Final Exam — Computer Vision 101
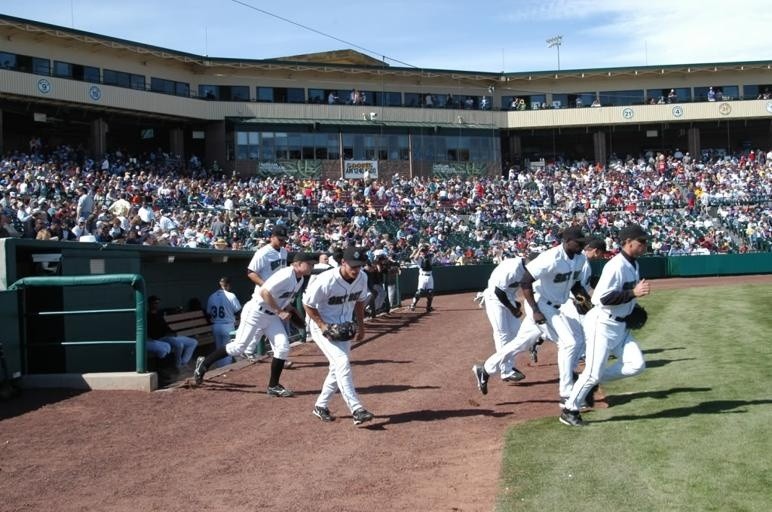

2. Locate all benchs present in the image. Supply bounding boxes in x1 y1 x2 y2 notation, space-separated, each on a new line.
148 309 240 352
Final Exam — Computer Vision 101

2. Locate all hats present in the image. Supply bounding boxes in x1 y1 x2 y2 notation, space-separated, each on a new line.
562 227 587 243
619 226 649 241
293 252 313 262
590 239 610 253
272 225 289 240
342 247 365 267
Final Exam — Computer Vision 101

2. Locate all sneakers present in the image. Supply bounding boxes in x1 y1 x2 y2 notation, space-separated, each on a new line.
558 397 588 412
502 370 522 382
353 408 373 425
473 364 489 395
411 303 415 309
312 406 332 421
558 408 584 427
529 337 544 363
266 384 293 397
426 308 432 313
194 356 207 383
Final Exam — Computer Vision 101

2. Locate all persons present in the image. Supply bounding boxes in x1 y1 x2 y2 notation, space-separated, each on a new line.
471 226 591 410
286 168 393 265
146 294 198 373
529 239 607 373
194 253 318 397
302 248 373 424
241 225 293 369
433 167 529 264
608 148 771 254
409 247 437 313
530 158 608 251
425 92 490 111
302 254 333 342
757 88 772 100
327 88 366 105
707 86 722 102
649 88 675 104
206 273 241 367
559 225 650 428
483 252 541 382
365 250 402 316
410 248 418 266
510 95 600 111
145 339 176 368
0 137 286 251
393 171 435 250
